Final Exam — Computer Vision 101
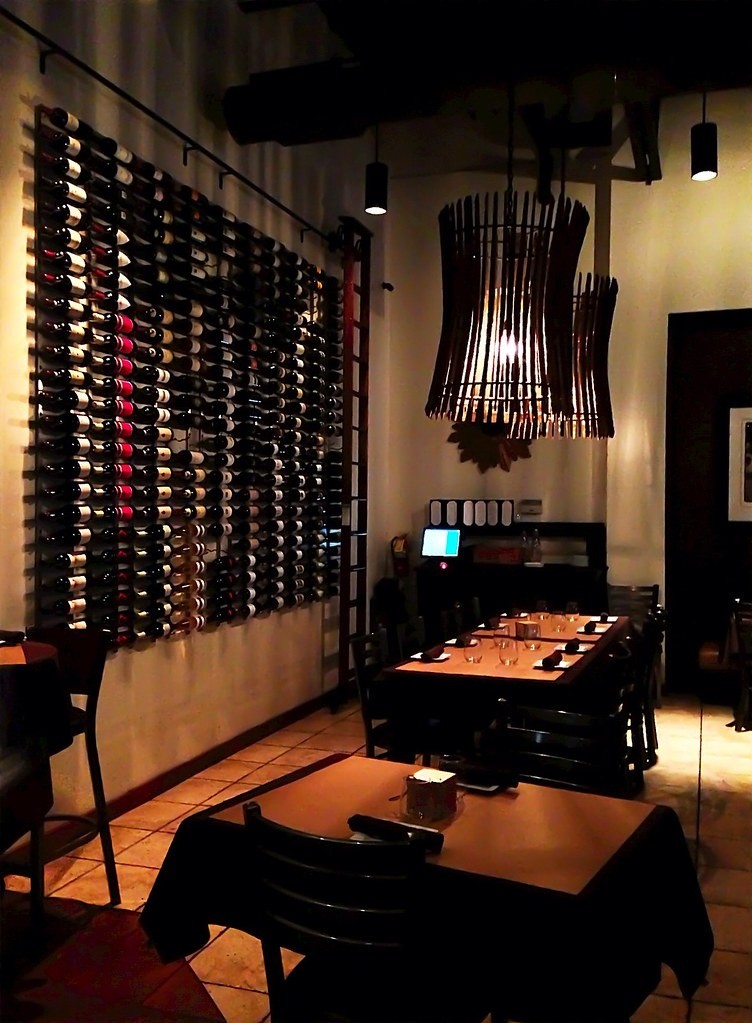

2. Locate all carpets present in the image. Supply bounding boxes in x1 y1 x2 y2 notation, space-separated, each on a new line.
0 885 227 1023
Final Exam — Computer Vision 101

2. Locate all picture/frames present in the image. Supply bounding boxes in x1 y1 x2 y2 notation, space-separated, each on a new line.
728 407 752 522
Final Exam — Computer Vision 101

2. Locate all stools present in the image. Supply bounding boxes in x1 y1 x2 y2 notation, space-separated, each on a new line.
1 627 118 918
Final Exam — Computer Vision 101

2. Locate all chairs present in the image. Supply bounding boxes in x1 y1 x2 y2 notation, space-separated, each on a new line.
350 584 669 801
242 802 488 1022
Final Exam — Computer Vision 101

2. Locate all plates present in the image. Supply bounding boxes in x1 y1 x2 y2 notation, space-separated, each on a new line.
533 659 570 668
590 616 618 622
445 639 477 645
411 652 451 660
553 644 587 652
500 613 527 617
478 623 508 628
577 626 606 633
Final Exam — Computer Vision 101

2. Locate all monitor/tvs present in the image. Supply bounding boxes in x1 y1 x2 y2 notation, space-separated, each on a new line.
420 527 461 559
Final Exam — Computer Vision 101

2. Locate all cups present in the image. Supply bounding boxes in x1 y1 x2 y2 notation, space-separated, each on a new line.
463 637 482 662
494 625 511 647
499 640 518 665
536 600 550 620
515 621 538 640
564 602 580 620
524 626 541 650
551 611 567 633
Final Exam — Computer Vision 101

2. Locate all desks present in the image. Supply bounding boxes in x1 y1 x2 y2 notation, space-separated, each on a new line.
720 599 752 732
0 642 77 936
139 753 717 1023
380 612 629 783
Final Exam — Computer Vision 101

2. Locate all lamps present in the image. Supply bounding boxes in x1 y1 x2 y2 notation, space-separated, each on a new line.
502 141 626 443
362 128 390 213
689 85 718 180
424 120 589 420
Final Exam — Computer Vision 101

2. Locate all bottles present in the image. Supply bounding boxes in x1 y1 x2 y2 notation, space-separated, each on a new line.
43 107 343 645
